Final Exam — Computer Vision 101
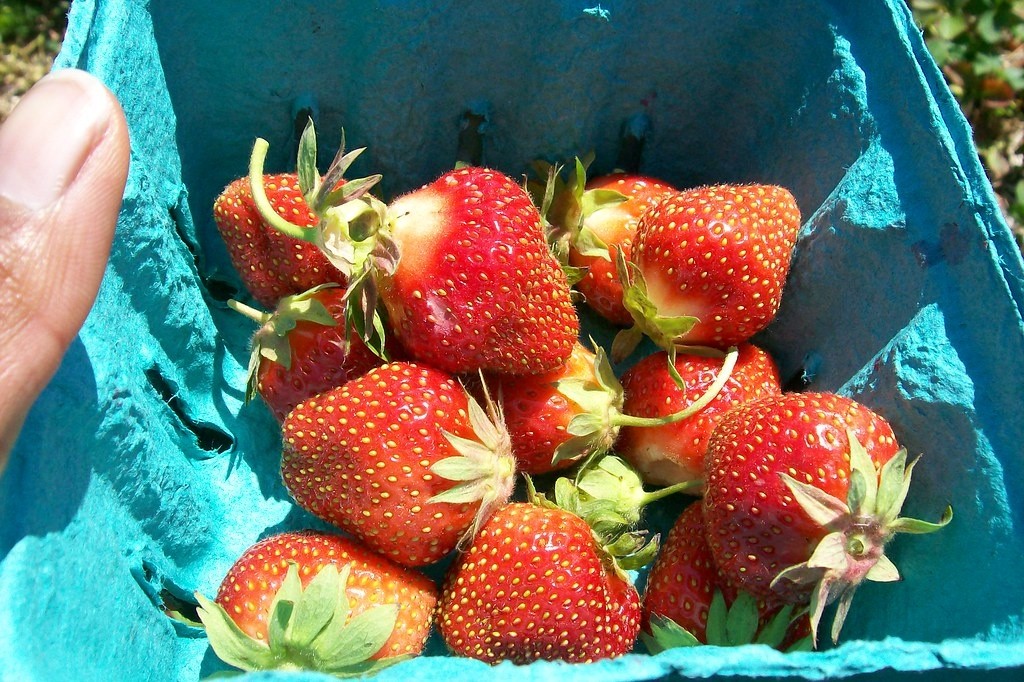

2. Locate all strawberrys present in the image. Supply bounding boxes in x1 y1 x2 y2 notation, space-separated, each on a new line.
187 116 956 681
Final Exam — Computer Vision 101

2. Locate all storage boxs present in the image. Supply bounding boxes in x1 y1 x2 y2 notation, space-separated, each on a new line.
0 0 1024 682
